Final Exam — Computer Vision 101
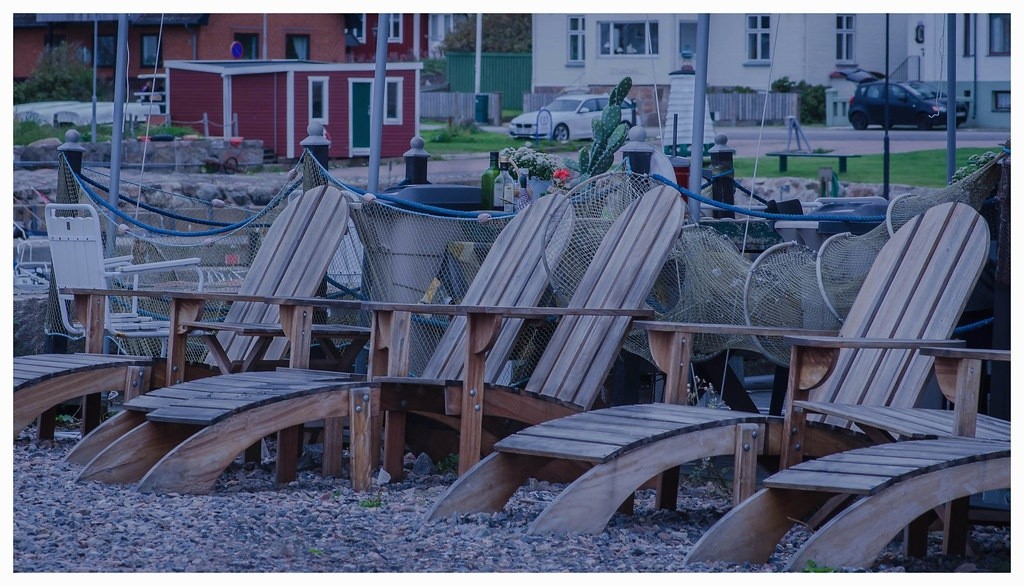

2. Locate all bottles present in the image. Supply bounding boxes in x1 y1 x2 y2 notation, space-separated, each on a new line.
515 172 531 213
480 151 500 210
492 162 514 214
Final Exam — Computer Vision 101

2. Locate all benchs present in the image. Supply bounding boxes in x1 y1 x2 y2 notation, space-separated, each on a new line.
765 147 863 174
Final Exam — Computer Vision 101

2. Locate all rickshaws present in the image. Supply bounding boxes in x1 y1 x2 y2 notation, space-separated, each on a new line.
200 152 247 176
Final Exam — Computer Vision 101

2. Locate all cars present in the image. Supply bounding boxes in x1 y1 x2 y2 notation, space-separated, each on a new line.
849 78 968 131
509 92 640 141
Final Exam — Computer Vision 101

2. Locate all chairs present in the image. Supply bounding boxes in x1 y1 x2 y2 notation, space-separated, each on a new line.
12 180 379 443
675 345 1012 574
421 199 996 539
42 201 211 357
59 191 576 488
137 180 690 506
